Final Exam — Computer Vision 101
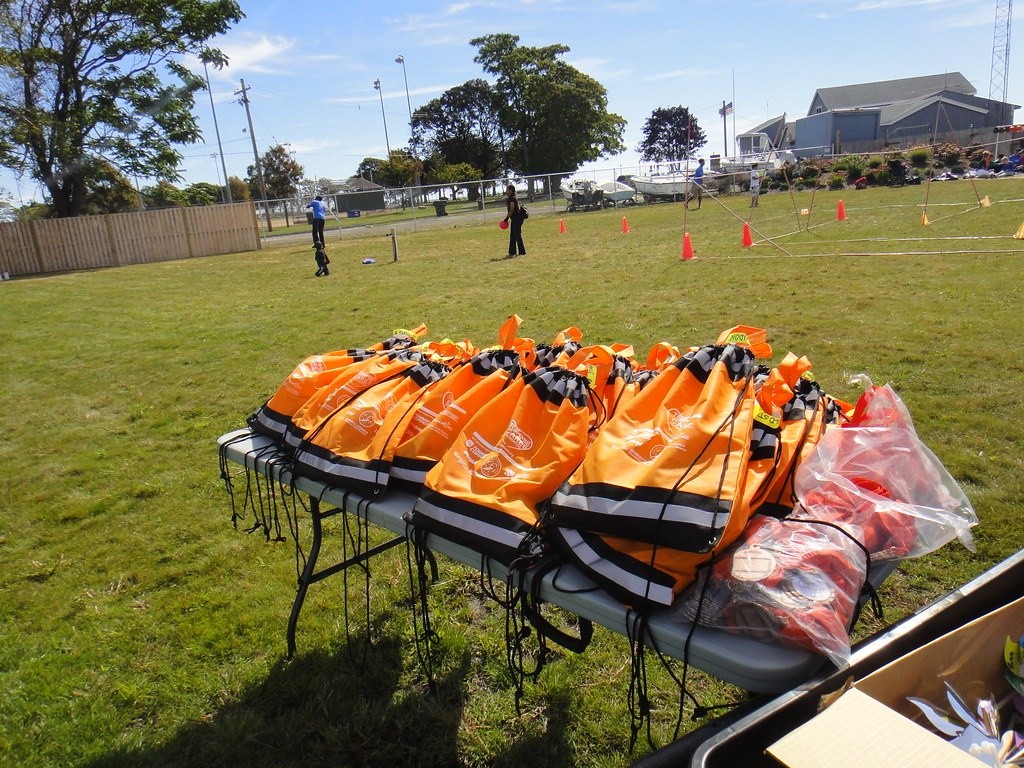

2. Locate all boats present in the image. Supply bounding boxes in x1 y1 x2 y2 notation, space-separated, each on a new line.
630 132 801 197
558 176 636 202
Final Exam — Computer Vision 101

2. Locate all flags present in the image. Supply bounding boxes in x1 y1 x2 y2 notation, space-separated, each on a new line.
721 102 732 118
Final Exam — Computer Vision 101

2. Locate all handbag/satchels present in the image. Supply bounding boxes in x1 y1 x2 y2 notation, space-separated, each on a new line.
248 325 846 615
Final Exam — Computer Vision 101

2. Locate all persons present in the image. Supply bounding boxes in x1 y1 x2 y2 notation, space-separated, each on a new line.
751 164 762 208
683 158 705 210
306 196 326 248
978 148 1024 179
501 185 527 258
315 241 330 277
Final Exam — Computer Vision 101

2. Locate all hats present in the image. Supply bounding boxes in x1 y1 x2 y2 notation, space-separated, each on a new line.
312 241 321 248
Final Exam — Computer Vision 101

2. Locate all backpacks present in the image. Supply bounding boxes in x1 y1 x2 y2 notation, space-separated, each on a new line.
509 197 529 220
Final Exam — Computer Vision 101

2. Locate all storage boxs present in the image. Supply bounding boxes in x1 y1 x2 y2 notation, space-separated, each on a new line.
690 545 1024 768
763 597 1024 768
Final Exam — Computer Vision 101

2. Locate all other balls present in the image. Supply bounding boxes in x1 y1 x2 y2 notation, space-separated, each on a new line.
500 221 509 230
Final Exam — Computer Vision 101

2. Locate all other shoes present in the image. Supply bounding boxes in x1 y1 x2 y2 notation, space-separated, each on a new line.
315 272 321 277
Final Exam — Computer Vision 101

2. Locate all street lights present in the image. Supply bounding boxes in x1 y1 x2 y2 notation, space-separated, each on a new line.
394 54 423 204
373 79 398 207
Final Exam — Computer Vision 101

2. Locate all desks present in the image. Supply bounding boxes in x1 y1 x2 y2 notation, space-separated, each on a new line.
217 427 896 702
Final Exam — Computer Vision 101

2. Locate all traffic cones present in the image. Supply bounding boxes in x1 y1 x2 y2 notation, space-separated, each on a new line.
834 198 849 222
619 216 630 234
559 218 567 234
741 222 757 248
679 232 697 261
